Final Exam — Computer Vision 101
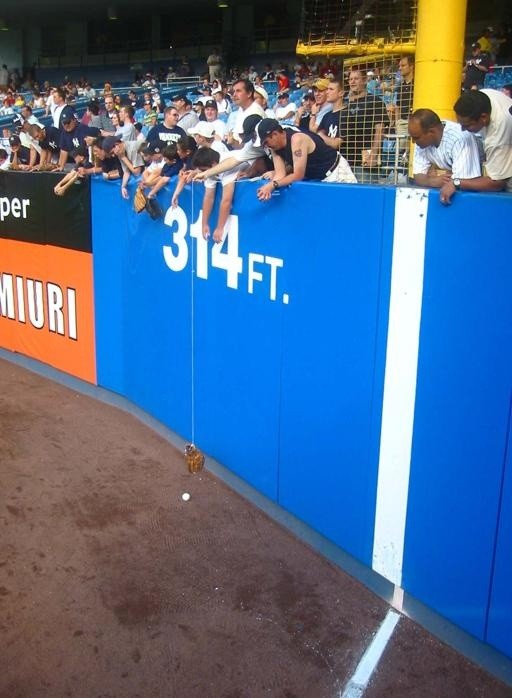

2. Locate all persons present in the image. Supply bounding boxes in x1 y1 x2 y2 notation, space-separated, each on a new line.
463 42 490 91
407 109 486 187
476 25 496 60
439 88 512 205
0 48 414 209
190 145 259 243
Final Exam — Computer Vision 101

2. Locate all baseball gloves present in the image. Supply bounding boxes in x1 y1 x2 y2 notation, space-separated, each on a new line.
39 162 59 171
146 197 160 220
185 444 205 475
9 162 26 170
133 188 146 214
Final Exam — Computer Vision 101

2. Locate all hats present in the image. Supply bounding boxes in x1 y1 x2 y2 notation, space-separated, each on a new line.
9 135 21 145
101 133 124 152
186 120 216 140
171 95 218 109
253 85 269 100
12 114 26 129
60 105 79 124
253 118 280 148
311 79 329 92
470 43 481 50
143 140 168 155
277 93 289 100
241 114 263 144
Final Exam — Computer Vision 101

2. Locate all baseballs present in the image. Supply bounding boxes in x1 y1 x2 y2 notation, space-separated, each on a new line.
181 493 191 500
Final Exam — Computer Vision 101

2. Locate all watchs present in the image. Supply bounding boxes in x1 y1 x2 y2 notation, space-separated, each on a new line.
453 178 461 190
